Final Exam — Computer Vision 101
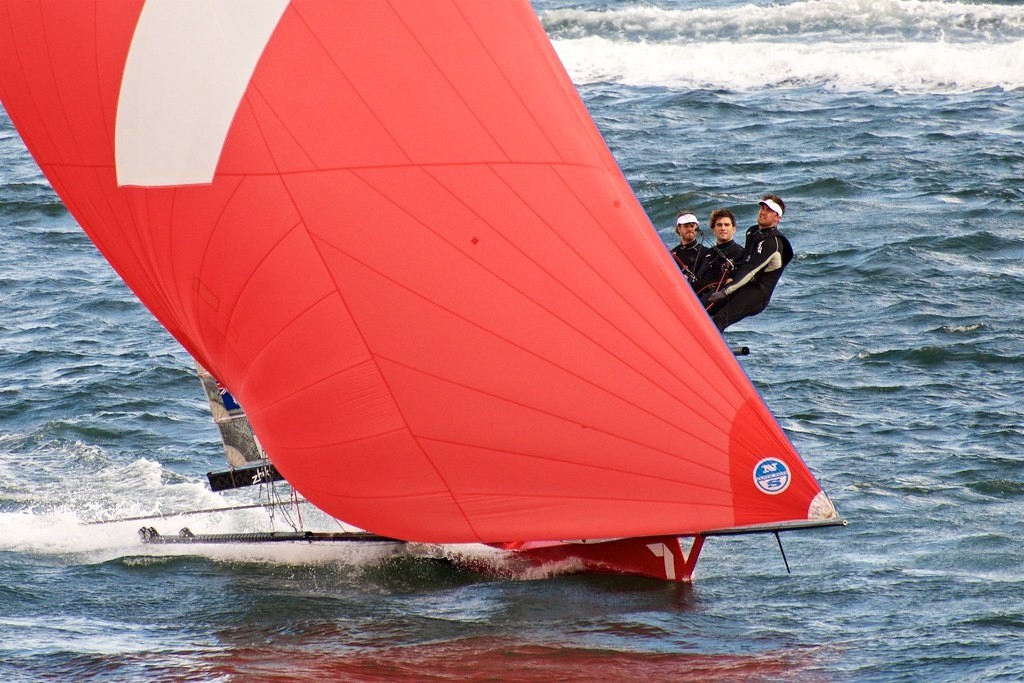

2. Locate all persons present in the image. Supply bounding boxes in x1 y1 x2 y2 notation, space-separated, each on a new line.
700 195 794 333
691 209 744 298
668 211 708 280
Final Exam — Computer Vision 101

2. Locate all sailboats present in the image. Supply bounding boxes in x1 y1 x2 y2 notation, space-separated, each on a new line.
0 0 848 592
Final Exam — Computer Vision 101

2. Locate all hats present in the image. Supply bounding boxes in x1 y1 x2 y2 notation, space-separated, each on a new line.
676 214 699 227
758 197 783 218
